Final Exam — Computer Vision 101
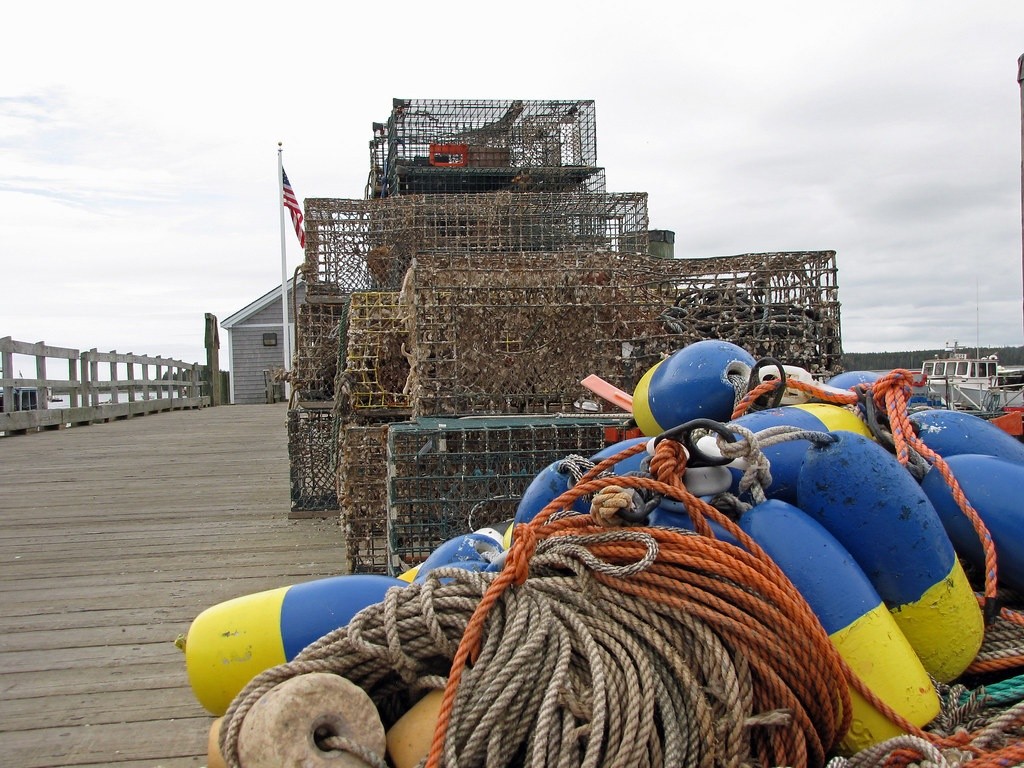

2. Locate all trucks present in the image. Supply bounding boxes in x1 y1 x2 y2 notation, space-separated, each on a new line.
0 386 49 412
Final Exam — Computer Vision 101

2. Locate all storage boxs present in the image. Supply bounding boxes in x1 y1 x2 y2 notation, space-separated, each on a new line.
283 97 842 574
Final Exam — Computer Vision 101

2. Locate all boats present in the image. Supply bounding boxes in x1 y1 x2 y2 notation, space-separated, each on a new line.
141 395 154 400
52 397 63 402
918 339 1024 409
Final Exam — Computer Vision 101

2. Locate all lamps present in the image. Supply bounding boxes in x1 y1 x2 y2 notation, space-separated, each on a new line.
263 333 278 346
